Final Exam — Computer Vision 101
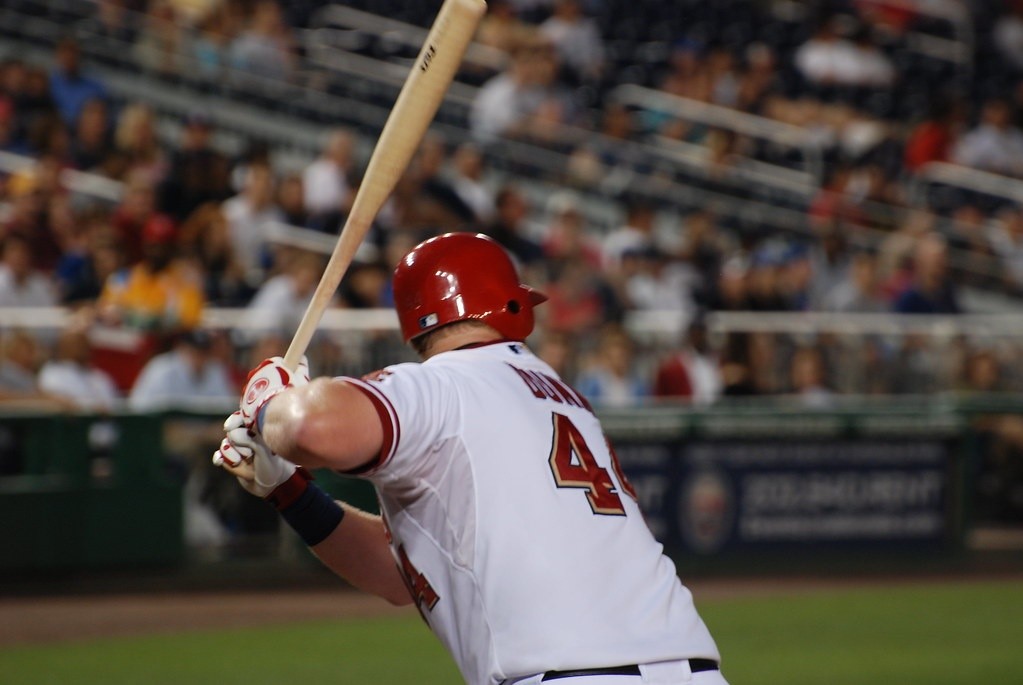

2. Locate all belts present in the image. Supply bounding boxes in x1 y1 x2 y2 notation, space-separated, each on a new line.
542 659 719 681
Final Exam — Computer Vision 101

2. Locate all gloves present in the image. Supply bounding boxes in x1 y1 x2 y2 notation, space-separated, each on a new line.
211 411 315 512
239 352 309 434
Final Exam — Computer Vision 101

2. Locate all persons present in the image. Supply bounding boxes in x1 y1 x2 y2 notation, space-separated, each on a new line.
0 0 1023 452
215 232 735 685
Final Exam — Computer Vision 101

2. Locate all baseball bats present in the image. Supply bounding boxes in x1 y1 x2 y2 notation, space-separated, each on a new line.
222 0 490 482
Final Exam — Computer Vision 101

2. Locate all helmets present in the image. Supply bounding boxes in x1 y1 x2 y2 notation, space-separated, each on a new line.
393 231 547 340
